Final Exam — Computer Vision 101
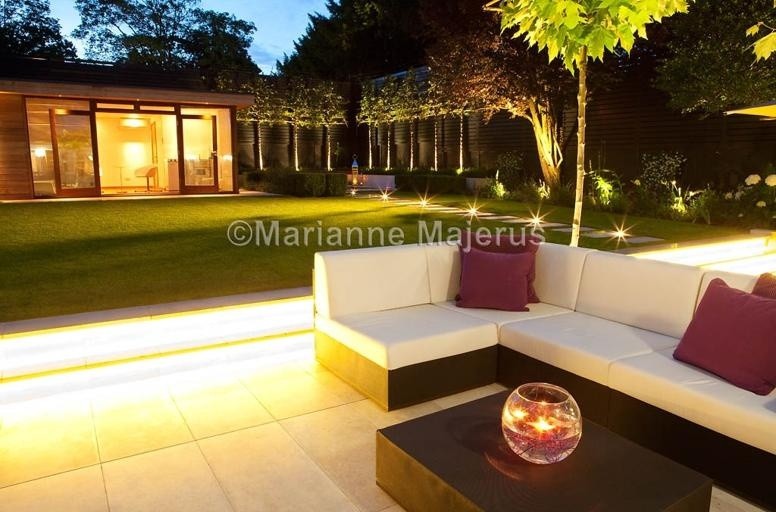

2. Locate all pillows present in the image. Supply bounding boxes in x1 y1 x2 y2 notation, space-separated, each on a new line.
455 230 541 303
673 279 776 395
751 273 776 298
456 244 534 312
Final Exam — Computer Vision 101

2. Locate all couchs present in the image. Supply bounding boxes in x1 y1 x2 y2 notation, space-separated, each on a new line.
427 239 776 512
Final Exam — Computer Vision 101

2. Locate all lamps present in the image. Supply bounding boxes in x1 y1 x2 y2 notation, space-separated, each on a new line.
351 154 359 185
501 382 583 465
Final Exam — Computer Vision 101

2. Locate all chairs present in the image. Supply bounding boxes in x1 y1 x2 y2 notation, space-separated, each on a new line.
134 165 162 192
313 244 498 412
